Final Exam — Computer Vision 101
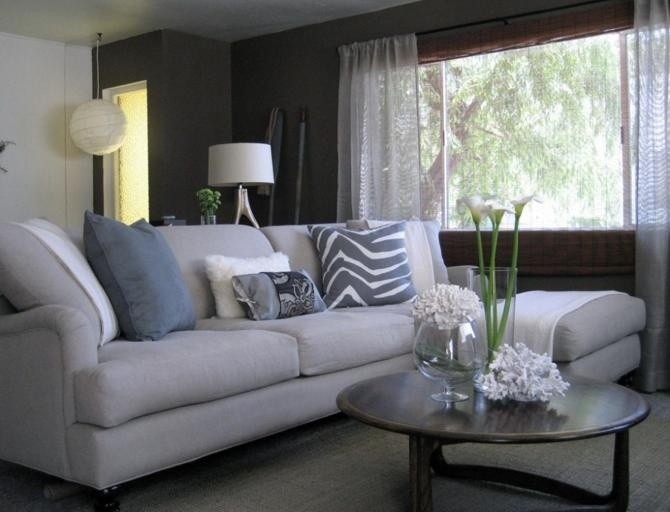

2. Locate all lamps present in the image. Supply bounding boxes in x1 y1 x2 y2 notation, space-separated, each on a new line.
67 30 130 158
209 143 275 228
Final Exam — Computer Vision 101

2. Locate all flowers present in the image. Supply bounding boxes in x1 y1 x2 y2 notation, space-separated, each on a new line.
194 187 221 224
457 192 545 373
474 342 570 406
410 281 480 333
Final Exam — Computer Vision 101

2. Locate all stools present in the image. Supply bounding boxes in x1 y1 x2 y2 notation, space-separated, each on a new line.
481 289 648 385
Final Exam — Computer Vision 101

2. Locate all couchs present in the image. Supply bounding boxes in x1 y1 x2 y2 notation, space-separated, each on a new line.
0 211 498 512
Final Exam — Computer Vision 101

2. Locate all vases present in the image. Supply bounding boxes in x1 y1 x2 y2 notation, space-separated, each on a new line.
506 389 537 403
467 268 517 390
200 215 217 225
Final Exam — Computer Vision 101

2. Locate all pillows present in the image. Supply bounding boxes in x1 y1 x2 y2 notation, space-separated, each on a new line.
84 212 198 340
200 251 291 319
0 215 120 350
231 269 328 320
306 222 419 310
